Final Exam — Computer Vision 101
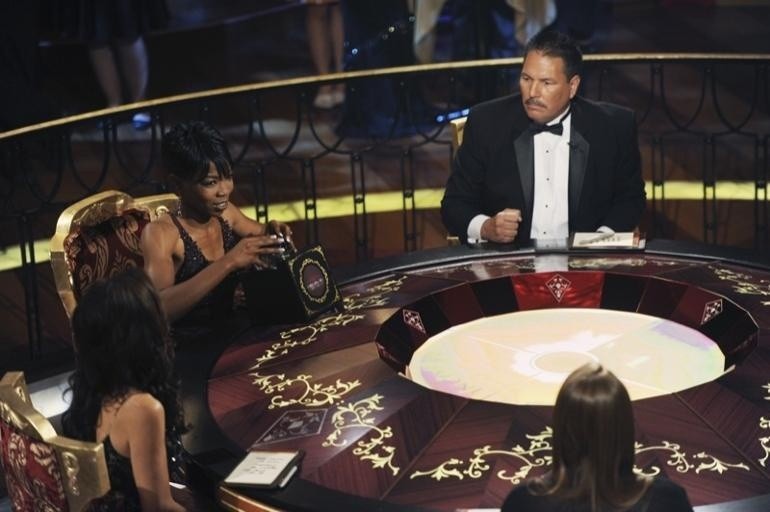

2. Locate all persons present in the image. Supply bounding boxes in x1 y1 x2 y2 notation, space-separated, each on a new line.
439 30 642 249
73 1 157 133
65 271 214 511
306 0 346 108
140 127 298 458
500 361 694 509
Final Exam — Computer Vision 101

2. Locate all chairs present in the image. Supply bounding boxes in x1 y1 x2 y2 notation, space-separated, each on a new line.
48 190 180 337
0 371 113 510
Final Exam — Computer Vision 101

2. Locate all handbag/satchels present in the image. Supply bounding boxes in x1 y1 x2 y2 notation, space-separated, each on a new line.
255 232 342 322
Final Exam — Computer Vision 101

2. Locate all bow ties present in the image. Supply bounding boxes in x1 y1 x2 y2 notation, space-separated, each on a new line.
528 121 564 137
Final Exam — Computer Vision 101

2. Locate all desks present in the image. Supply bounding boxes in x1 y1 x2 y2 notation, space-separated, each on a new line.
177 238 768 512
